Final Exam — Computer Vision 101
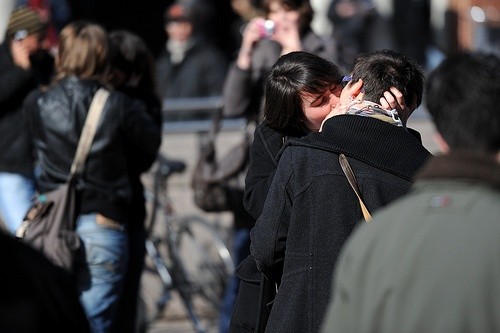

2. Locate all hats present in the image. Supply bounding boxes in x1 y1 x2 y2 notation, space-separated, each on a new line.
164 0 198 22
7 5 46 41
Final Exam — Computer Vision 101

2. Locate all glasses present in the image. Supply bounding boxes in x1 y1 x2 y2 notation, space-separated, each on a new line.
341 75 364 93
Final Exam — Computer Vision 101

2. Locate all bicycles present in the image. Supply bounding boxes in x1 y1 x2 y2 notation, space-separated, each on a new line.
141 153 237 333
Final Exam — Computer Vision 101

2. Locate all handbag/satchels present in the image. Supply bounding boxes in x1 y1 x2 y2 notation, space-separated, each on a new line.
192 105 254 212
14 171 83 275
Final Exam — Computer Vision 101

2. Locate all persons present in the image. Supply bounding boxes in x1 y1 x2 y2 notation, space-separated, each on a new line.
221 1 328 132
321 47 500 332
0 1 57 241
146 1 235 102
250 50 438 332
1 214 95 333
230 50 414 331
13 17 165 332
